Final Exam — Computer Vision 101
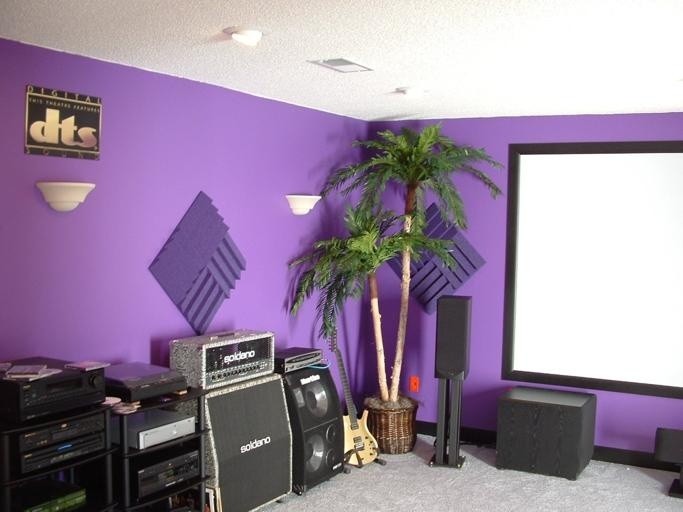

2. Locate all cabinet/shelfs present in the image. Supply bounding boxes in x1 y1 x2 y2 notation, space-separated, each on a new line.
0 386 212 512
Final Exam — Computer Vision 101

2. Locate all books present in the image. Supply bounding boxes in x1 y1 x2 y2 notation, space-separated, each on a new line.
1 368 63 382
195 483 222 512
4 363 47 378
63 358 111 371
165 481 213 512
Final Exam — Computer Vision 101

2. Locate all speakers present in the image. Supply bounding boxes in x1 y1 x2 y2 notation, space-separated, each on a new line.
436 294 472 376
202 375 293 512
283 361 344 494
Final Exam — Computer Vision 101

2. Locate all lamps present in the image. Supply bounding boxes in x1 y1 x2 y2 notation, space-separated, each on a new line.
36 182 96 212
285 195 322 215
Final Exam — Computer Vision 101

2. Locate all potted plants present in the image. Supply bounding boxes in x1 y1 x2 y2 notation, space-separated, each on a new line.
288 121 506 454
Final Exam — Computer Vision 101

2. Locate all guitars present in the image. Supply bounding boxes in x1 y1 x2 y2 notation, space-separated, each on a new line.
330 329 378 466
